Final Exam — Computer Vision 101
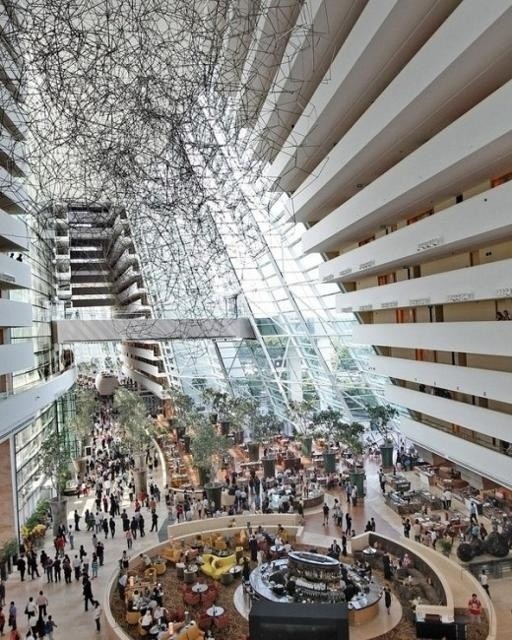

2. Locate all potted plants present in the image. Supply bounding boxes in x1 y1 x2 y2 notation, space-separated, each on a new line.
289 396 399 500
173 388 276 511
42 427 76 536
116 415 161 501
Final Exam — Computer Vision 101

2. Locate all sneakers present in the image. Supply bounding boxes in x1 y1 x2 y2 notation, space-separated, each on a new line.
322 522 329 526
91 575 98 580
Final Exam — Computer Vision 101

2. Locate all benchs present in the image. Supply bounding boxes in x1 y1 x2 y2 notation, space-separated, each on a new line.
360 546 441 607
198 550 239 583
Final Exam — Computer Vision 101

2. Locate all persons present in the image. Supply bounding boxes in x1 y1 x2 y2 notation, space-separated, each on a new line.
10 253 22 262
496 310 510 321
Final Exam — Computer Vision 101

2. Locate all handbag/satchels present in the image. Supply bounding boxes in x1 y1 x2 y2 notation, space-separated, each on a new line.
9 618 12 626
25 607 28 614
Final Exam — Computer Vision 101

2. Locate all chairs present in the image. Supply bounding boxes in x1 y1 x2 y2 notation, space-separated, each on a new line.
241 575 257 609
123 567 233 640
